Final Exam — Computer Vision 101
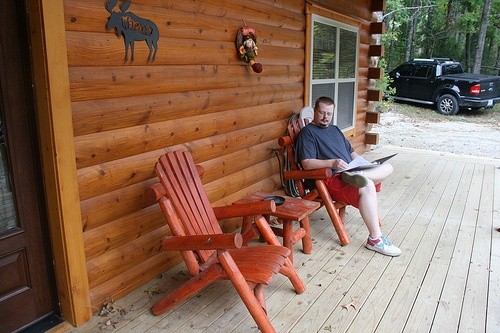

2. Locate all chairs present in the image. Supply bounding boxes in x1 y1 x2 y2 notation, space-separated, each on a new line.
277 117 383 246
146 149 306 332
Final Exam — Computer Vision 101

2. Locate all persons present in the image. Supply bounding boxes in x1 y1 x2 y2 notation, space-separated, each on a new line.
295 96 402 257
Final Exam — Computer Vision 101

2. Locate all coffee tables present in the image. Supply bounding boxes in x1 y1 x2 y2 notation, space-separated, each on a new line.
231 190 321 264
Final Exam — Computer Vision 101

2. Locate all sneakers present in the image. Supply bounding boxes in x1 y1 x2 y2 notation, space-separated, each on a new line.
365 234 402 256
342 170 369 189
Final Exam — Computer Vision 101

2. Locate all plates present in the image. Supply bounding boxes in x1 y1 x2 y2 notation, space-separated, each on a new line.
264 196 285 206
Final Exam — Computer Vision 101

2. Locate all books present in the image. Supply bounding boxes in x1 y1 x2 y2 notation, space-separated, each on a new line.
335 152 398 173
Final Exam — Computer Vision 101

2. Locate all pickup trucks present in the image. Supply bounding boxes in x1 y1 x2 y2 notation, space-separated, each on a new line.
384 58 500 115
